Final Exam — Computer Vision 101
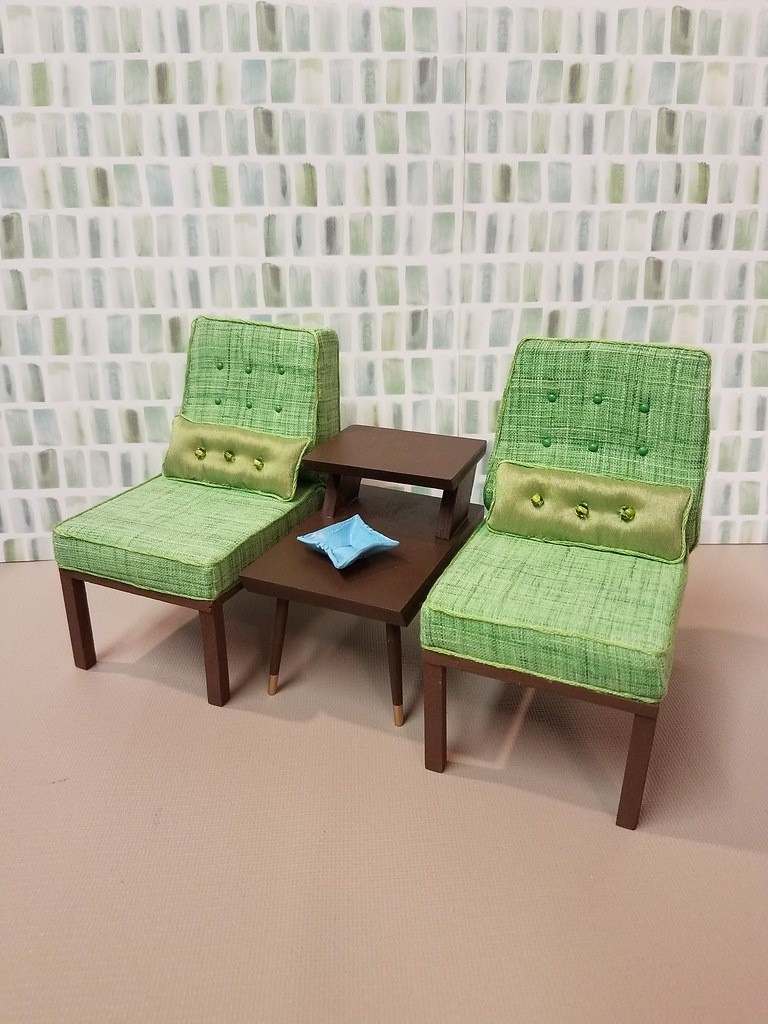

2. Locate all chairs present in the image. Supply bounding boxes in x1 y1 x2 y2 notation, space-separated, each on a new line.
417 333 715 832
48 311 345 710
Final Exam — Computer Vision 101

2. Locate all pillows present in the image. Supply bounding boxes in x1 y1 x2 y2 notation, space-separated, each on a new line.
486 457 694 566
162 410 314 504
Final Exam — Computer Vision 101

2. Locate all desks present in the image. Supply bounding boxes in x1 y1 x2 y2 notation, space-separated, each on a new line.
236 424 491 730
